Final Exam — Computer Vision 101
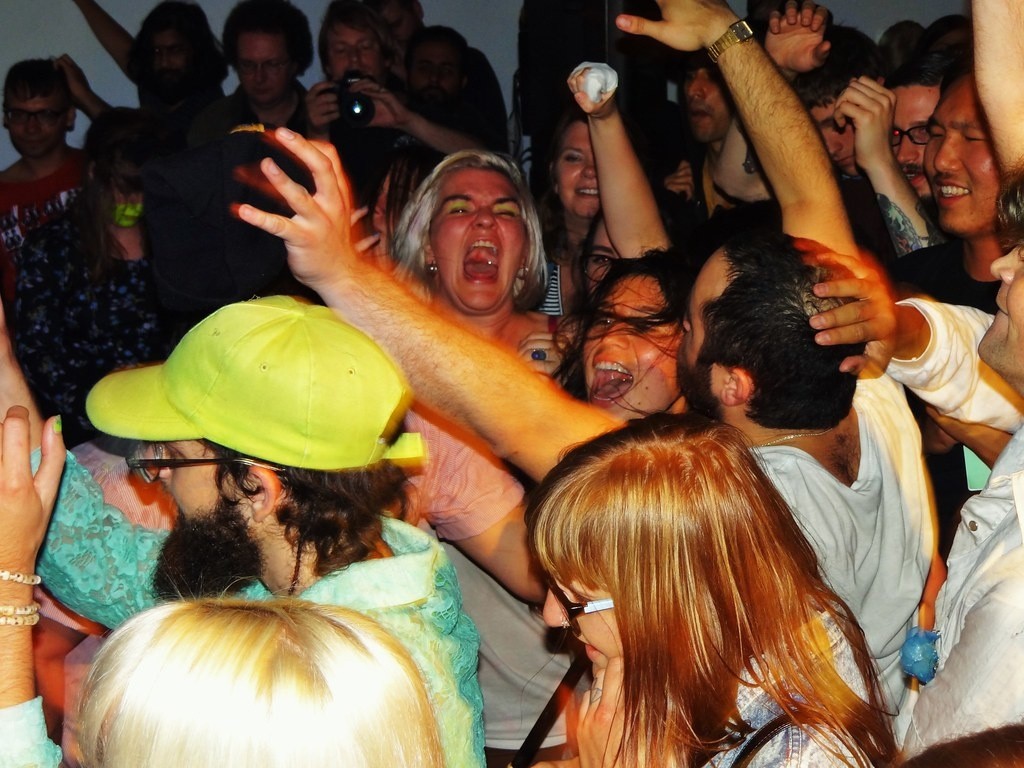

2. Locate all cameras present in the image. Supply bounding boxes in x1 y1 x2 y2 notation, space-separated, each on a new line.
317 69 382 128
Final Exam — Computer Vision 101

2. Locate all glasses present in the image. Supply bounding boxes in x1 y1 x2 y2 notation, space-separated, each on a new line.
120 440 281 484
576 252 615 282
2 105 66 128
889 121 934 151
543 574 616 622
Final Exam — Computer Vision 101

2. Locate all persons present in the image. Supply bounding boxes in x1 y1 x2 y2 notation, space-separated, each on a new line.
2 2 1024 767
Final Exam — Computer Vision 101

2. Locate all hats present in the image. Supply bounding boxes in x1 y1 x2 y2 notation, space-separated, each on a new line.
83 290 426 473
80 104 186 205
